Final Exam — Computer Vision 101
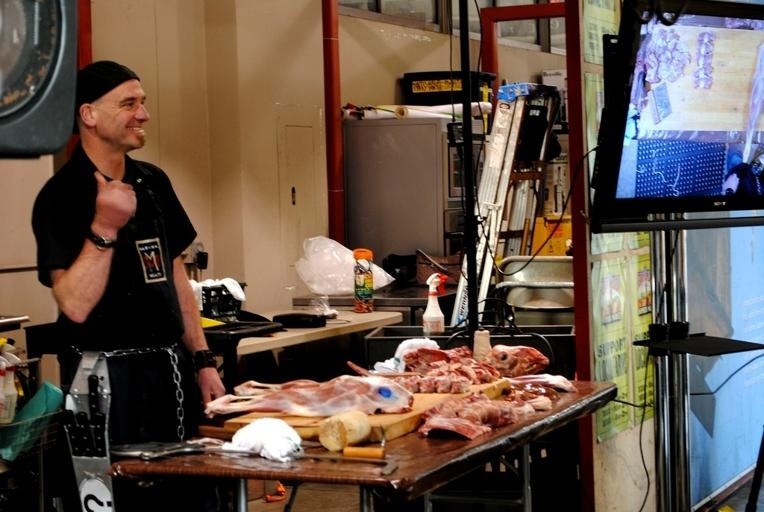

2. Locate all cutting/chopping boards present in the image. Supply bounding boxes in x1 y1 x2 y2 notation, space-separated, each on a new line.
224 379 511 441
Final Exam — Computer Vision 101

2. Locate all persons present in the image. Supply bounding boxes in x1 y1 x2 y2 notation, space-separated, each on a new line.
31 60 227 512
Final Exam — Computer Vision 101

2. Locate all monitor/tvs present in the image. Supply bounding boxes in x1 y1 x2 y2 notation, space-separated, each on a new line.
590 0 764 234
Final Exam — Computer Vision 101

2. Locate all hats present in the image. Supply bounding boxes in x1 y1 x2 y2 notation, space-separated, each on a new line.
74 62 140 133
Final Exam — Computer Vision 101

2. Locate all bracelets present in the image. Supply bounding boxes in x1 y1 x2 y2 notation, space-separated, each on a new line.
193 351 218 374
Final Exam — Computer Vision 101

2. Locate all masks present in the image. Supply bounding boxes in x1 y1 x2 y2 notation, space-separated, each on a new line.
222 416 302 463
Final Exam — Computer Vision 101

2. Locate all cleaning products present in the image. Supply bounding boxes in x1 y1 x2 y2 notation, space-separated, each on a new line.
0 338 20 424
423 272 448 332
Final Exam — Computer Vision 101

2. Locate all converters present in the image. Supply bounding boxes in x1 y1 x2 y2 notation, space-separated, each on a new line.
197 252 208 269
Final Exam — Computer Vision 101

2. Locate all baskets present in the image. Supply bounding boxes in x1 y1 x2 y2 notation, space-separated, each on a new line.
201 283 247 323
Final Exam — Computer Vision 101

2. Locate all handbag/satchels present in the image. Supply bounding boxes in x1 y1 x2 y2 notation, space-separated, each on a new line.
518 104 561 161
387 254 416 281
416 248 461 286
382 260 411 290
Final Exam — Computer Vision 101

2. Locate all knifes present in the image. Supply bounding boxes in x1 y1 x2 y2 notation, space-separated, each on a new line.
62 375 106 460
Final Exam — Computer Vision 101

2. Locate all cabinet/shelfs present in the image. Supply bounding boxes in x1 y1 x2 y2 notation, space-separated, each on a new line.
107 338 617 512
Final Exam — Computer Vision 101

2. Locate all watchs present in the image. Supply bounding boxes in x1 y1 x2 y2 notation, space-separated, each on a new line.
86 228 118 251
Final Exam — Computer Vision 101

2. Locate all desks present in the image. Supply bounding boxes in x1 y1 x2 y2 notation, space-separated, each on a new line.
204 308 403 392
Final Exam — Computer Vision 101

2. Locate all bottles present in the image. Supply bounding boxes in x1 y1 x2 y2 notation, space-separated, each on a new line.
354 249 374 313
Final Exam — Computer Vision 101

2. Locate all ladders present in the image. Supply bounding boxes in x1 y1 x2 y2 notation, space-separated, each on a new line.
450 83 556 328
